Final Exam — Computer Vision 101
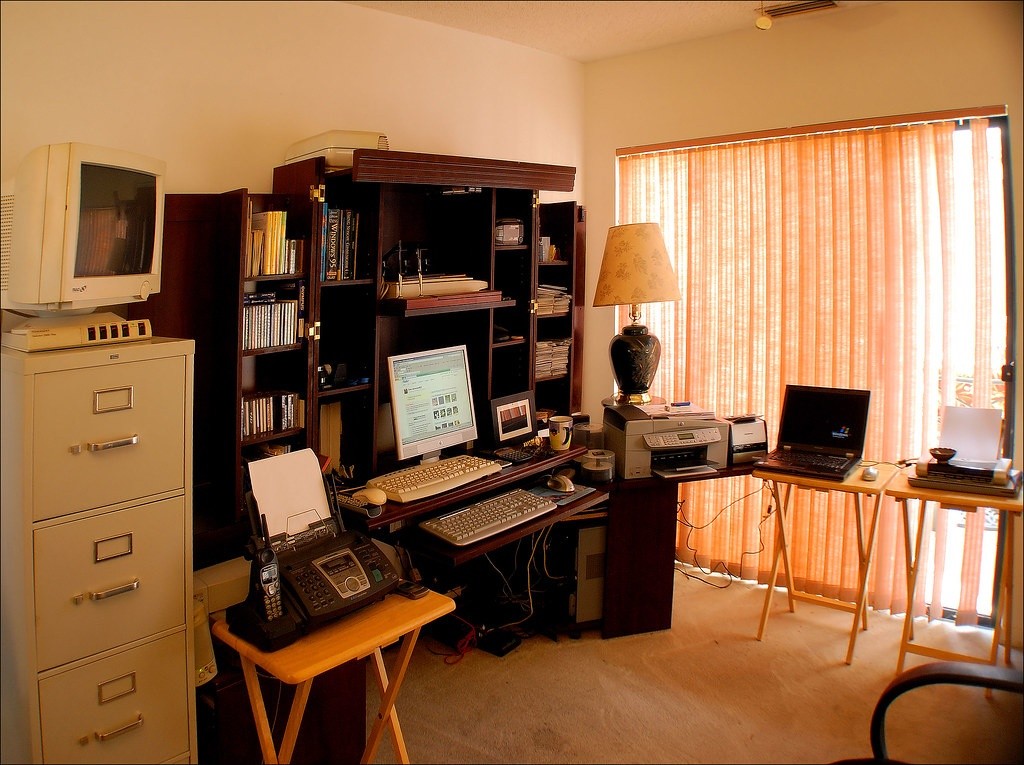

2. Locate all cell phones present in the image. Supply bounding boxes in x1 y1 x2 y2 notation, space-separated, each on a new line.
394 579 430 600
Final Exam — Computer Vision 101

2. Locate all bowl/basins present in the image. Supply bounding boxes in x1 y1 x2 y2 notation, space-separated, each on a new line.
929 448 957 463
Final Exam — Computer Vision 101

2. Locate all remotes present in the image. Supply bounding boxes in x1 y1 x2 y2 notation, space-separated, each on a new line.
330 494 383 518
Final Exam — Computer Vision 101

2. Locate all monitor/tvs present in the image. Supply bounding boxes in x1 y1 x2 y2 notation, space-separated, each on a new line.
0 141 166 353
387 345 478 465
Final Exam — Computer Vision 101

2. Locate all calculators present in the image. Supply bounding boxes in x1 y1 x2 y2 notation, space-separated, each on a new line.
493 447 533 463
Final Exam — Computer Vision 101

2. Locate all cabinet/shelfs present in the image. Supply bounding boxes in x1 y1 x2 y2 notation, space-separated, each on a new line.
601 464 757 639
202 145 585 565
0 335 199 765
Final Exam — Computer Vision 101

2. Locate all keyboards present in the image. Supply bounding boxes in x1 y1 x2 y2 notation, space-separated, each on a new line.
365 454 503 503
418 487 558 547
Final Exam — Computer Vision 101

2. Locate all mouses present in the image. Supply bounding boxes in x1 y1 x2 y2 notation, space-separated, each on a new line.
862 467 879 481
546 475 575 493
352 489 387 506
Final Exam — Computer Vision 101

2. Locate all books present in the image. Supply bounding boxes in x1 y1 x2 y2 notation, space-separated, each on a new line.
245 196 297 278
241 392 304 441
537 284 572 315
320 203 359 282
536 337 571 378
243 279 305 350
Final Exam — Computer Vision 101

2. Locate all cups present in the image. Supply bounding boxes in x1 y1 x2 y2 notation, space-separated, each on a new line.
571 412 590 425
549 416 573 450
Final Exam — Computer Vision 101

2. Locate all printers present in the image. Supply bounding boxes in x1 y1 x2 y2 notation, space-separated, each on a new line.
603 403 730 478
916 418 1013 486
716 413 768 466
244 475 399 634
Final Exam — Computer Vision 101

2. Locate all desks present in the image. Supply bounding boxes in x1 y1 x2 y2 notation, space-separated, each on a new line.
342 442 609 657
752 462 900 667
210 590 458 765
884 464 1024 698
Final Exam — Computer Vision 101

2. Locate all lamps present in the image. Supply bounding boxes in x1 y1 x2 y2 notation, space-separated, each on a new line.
593 221 685 407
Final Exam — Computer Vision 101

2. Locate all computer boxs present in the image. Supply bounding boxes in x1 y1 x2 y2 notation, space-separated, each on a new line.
546 525 603 624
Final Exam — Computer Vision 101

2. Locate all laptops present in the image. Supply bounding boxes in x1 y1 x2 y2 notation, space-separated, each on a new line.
752 385 870 480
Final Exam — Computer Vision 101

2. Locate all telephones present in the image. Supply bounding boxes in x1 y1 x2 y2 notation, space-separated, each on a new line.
224 514 296 653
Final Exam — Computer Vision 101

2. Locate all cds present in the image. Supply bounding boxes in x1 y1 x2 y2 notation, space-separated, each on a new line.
583 461 613 470
